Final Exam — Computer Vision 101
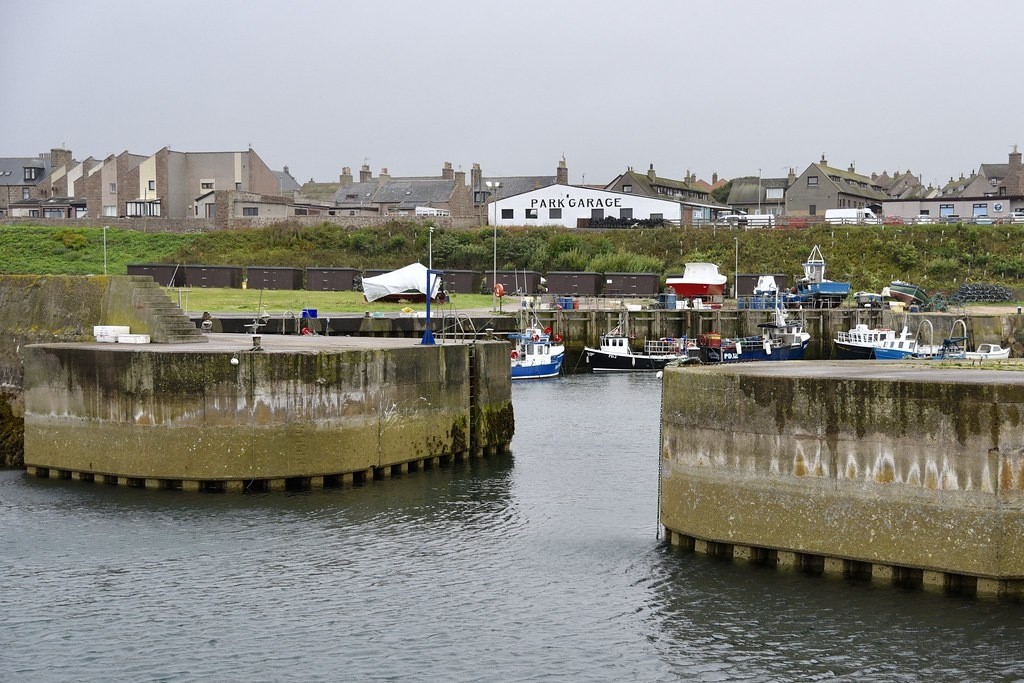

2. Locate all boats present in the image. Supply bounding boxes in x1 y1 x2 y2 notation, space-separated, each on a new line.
360 262 443 305
508 267 566 379
853 286 895 309
705 287 811 364
833 323 921 360
871 318 1014 360
889 280 930 306
736 275 802 310
794 243 851 307
584 304 704 374
665 262 728 310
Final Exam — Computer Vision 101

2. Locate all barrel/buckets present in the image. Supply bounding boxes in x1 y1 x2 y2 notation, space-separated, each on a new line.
557 298 579 310
739 297 782 309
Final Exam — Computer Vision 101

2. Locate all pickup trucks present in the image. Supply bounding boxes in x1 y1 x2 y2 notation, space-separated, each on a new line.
1002 208 1024 224
970 214 998 225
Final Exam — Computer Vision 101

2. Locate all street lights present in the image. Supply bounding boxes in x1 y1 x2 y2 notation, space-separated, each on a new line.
103 225 111 276
429 225 435 271
486 180 505 316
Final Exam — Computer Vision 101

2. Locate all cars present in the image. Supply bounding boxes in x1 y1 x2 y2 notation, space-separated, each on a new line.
938 214 963 226
884 215 904 225
909 214 936 225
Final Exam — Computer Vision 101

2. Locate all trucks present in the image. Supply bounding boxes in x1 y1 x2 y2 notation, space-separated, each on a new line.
825 207 883 225
714 214 776 230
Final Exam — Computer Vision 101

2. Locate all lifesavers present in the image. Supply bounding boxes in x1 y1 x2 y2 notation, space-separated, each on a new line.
688 342 696 347
495 284 503 297
699 335 707 346
881 328 891 331
